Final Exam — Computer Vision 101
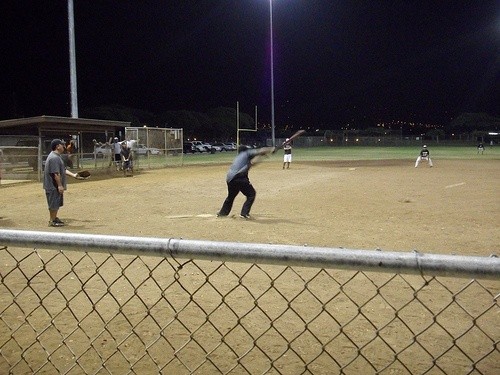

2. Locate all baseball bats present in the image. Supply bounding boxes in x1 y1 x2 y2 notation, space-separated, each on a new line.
273 129 304 153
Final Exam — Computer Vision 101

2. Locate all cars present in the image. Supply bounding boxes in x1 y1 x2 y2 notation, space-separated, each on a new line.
93 142 161 159
182 141 258 154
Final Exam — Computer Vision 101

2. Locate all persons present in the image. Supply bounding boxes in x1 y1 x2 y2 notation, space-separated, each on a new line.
283 139 292 169
415 145 432 168
477 143 485 154
217 146 275 218
43 136 140 226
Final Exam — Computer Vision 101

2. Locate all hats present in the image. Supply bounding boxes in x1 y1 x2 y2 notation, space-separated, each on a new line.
114 137 119 140
51 139 66 147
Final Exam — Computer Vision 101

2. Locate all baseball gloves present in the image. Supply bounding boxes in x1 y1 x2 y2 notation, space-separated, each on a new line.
74 170 92 180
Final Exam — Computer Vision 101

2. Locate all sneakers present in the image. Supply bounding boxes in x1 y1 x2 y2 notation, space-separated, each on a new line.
216 213 223 219
240 214 252 219
48 217 65 228
